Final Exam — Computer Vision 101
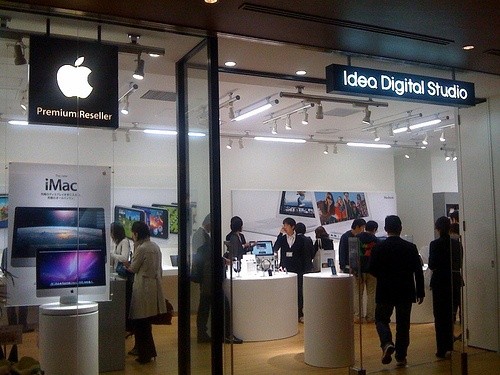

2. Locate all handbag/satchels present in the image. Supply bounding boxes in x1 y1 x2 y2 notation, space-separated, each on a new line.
313 238 322 272
150 299 173 325
115 261 132 275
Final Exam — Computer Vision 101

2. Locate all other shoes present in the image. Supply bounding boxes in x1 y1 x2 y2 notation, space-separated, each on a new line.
298 316 304 322
436 351 451 360
397 356 407 366
353 318 363 324
225 336 243 344
197 335 211 343
367 317 374 323
127 348 137 356
381 344 395 364
22 326 35 333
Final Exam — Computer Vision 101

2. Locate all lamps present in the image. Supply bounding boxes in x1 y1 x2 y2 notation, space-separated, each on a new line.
315 104 324 120
112 131 118 142
285 115 293 131
444 151 457 161
239 138 244 151
388 125 395 137
228 105 234 120
302 109 308 125
126 133 130 142
375 135 380 141
226 137 232 149
405 120 412 134
361 109 372 125
323 145 328 154
13 38 26 65
440 131 445 142
422 135 429 145
234 99 280 122
133 52 145 80
404 152 415 159
272 119 277 135
121 98 129 115
333 143 338 155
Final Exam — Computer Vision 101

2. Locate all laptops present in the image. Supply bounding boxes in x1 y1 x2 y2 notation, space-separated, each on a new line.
242 191 317 235
311 192 387 240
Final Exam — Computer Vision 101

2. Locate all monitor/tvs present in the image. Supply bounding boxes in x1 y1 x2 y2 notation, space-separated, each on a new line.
35 247 106 305
10 206 107 266
249 240 274 256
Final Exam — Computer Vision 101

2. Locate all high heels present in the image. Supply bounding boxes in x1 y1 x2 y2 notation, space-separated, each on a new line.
135 353 157 363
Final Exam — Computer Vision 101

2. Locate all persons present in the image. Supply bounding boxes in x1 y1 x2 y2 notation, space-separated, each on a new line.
317 192 368 226
429 216 465 359
225 216 258 260
364 221 381 322
296 191 307 207
110 221 134 338
339 219 369 324
370 215 426 367
123 220 173 365
313 226 335 268
190 213 244 344
0 247 35 333
273 218 315 323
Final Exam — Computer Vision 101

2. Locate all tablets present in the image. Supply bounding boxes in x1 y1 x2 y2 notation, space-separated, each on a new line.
170 255 178 266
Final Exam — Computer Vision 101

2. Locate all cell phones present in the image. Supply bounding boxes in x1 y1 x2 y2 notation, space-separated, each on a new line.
330 266 337 276
114 204 179 241
268 269 272 277
